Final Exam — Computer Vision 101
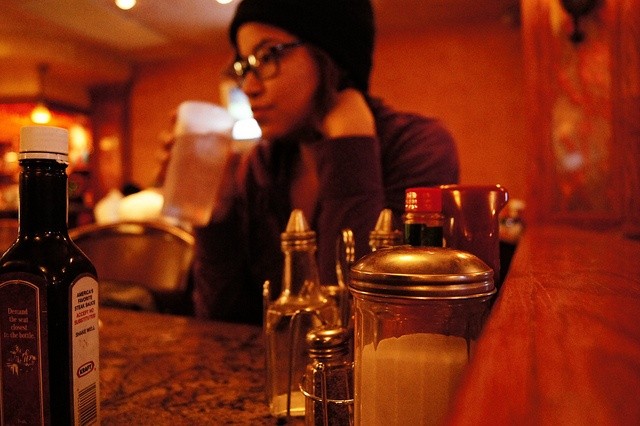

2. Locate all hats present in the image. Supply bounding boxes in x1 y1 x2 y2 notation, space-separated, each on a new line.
229 0 375 89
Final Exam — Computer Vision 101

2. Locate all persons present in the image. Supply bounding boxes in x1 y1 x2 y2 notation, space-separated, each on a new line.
190 0 460 327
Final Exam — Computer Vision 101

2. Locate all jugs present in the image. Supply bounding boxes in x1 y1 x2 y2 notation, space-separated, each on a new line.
344 245 498 426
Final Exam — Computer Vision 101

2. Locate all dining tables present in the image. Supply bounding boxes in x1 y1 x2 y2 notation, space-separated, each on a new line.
98 305 272 426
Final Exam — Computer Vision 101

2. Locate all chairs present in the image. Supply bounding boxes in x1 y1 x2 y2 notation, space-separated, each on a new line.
70 218 196 316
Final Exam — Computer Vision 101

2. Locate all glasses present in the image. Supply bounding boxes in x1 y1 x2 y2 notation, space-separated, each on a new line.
227 36 301 81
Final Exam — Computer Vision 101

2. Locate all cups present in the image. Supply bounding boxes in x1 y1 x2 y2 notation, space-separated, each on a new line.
160 101 237 225
438 186 508 282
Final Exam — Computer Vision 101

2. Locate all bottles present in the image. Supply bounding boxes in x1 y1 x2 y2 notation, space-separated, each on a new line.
267 209 336 418
370 209 402 251
405 189 443 247
0 127 99 426
304 325 354 426
